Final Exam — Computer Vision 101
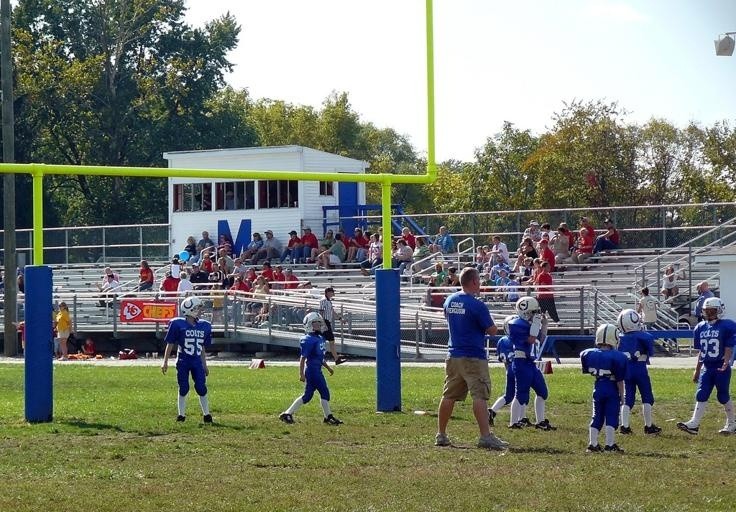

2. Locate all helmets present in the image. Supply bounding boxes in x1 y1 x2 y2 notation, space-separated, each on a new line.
701 297 726 321
595 323 621 349
303 312 328 332
515 297 541 321
503 314 518 338
180 296 205 320
617 309 645 332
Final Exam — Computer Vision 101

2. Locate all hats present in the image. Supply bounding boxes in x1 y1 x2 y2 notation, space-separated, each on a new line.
192 263 199 266
530 216 613 244
325 287 336 292
264 225 311 235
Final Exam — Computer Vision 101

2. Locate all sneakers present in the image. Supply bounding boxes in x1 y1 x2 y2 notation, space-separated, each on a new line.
278 412 295 424
336 358 347 366
323 414 343 425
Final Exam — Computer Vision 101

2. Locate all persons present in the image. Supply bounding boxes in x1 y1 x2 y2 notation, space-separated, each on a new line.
507 294 558 431
277 310 345 426
675 296 736 437
225 191 240 209
662 266 687 299
638 286 661 330
580 322 629 453
158 225 320 328
0 259 156 361
487 313 536 427
310 226 454 284
424 217 621 325
434 266 511 449
695 282 702 295
159 295 213 424
615 308 664 435
317 288 347 365
194 191 211 209
694 279 716 323
